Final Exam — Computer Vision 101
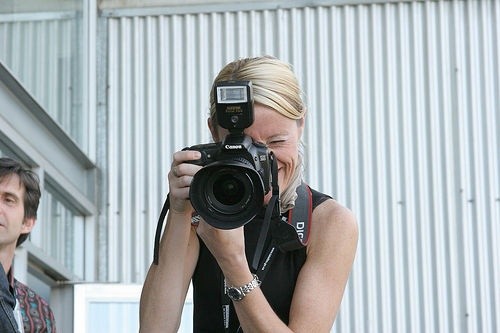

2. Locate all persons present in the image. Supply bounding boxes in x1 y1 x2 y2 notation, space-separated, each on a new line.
136 53 360 332
0 156 59 333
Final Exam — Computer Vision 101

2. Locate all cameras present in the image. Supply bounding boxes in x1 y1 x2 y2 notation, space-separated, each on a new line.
180 80 273 231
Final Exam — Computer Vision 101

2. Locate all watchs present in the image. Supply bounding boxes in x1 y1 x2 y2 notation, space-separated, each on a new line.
225 272 261 300
